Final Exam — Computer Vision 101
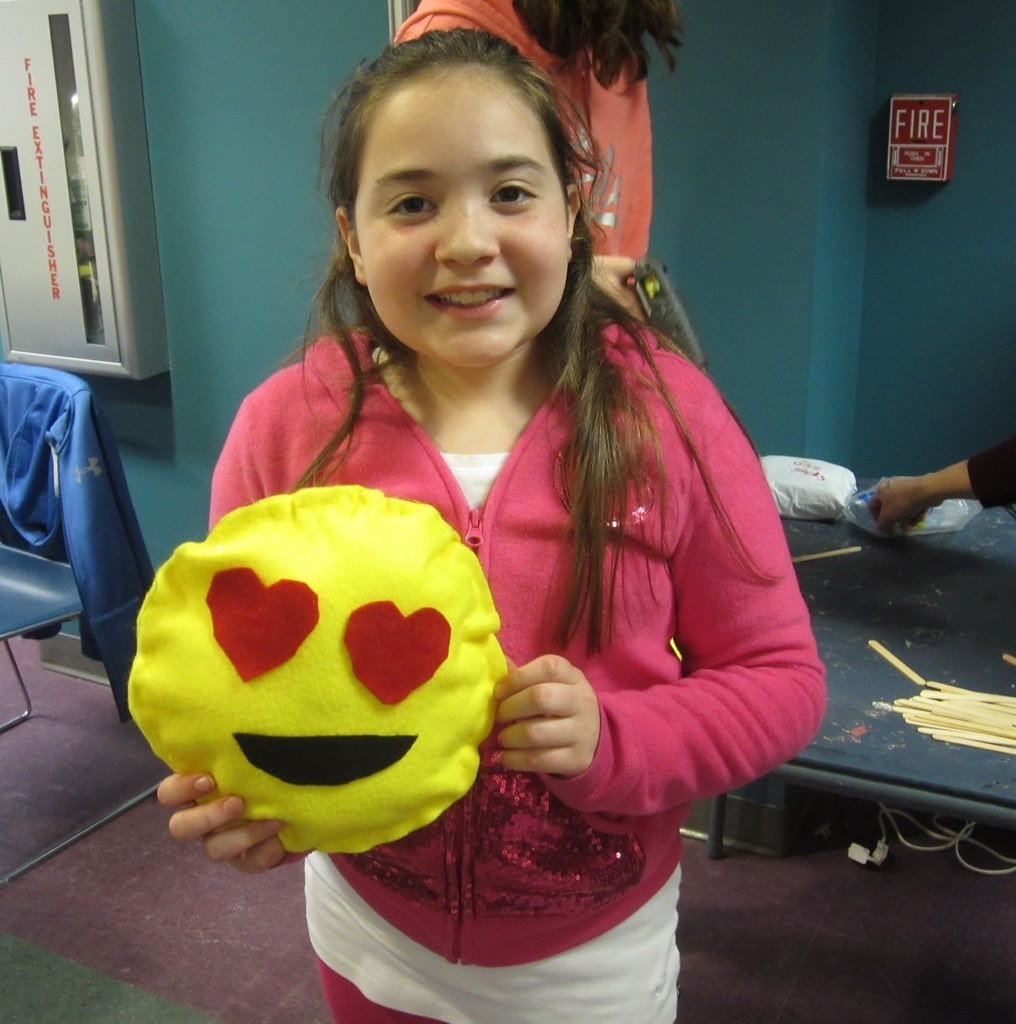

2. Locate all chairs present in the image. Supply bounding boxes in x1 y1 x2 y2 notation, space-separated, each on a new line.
0 362 157 737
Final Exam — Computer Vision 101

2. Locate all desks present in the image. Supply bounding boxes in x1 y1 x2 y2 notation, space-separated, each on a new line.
704 456 1016 857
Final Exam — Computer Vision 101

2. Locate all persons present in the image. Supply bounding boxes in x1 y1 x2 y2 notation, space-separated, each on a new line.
158 28 828 1023
392 0 683 324
867 436 1016 534
